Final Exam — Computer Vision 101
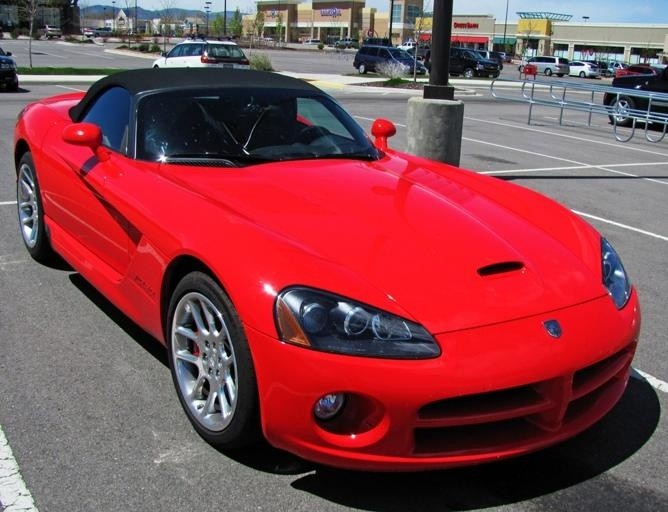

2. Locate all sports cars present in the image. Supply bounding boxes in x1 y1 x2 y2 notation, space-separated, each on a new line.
12 67 640 481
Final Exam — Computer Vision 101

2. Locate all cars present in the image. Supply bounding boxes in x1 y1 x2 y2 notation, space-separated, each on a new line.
353 41 429 74
335 38 359 48
40 24 62 38
0 46 19 92
151 40 251 70
604 61 667 132
426 47 503 78
83 26 110 36
520 55 599 78
